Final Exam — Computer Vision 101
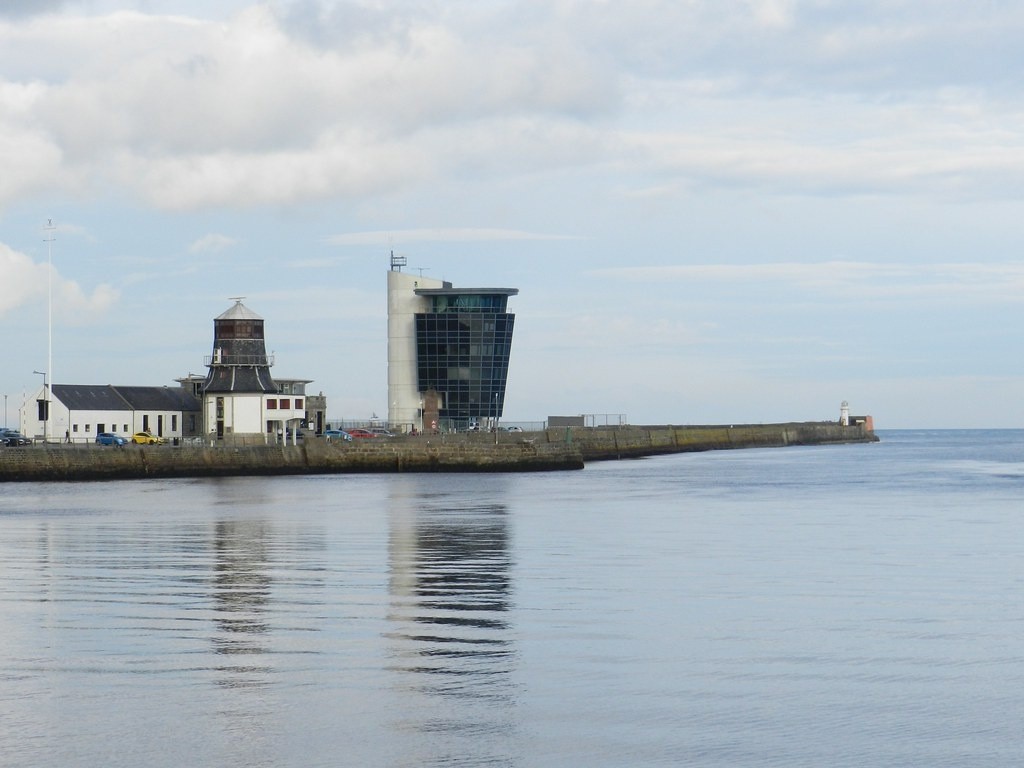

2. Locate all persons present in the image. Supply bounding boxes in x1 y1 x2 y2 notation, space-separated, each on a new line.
209 429 217 448
147 428 152 436
65 429 70 442
339 426 342 432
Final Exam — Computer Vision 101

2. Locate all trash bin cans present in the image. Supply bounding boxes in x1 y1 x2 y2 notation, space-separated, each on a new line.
174 437 179 445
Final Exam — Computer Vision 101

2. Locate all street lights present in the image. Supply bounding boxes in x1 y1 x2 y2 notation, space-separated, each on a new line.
419 400 423 435
4 394 8 428
392 402 396 428
33 371 46 443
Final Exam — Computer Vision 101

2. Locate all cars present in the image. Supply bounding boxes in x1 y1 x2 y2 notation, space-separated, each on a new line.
96 432 128 445
322 429 355 442
474 426 490 433
277 428 305 440
369 428 396 437
508 426 522 432
491 427 507 432
409 429 421 436
348 429 377 438
0 428 32 446
131 432 165 445
